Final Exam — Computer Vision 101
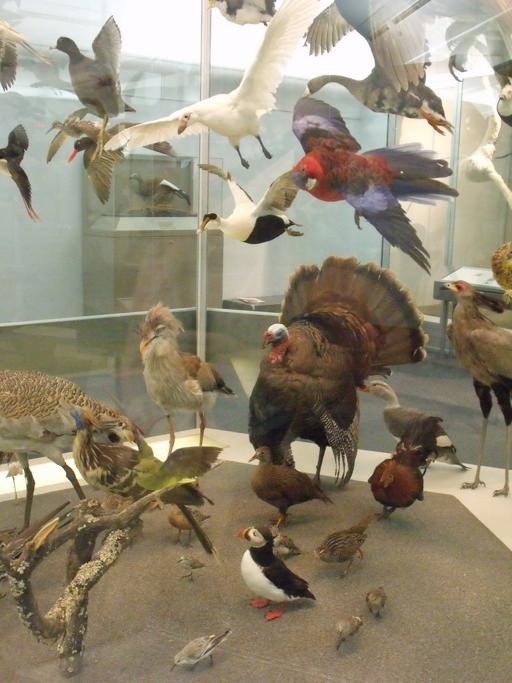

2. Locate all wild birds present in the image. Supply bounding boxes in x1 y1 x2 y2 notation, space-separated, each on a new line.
441 274 511 497
240 261 468 655
0 302 234 682
5 2 459 262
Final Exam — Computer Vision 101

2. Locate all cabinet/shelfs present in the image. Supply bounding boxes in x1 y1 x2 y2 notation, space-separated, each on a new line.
81 152 224 315
